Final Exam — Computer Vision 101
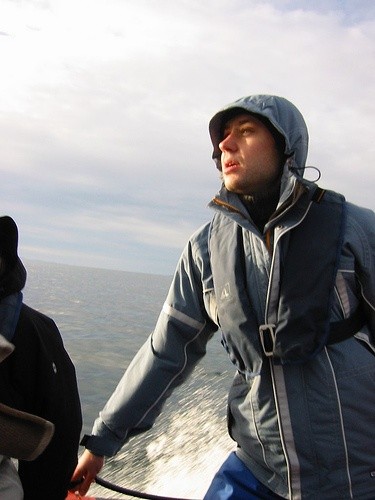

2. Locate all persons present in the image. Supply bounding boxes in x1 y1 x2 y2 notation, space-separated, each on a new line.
72 93 375 500
0 214 83 500
